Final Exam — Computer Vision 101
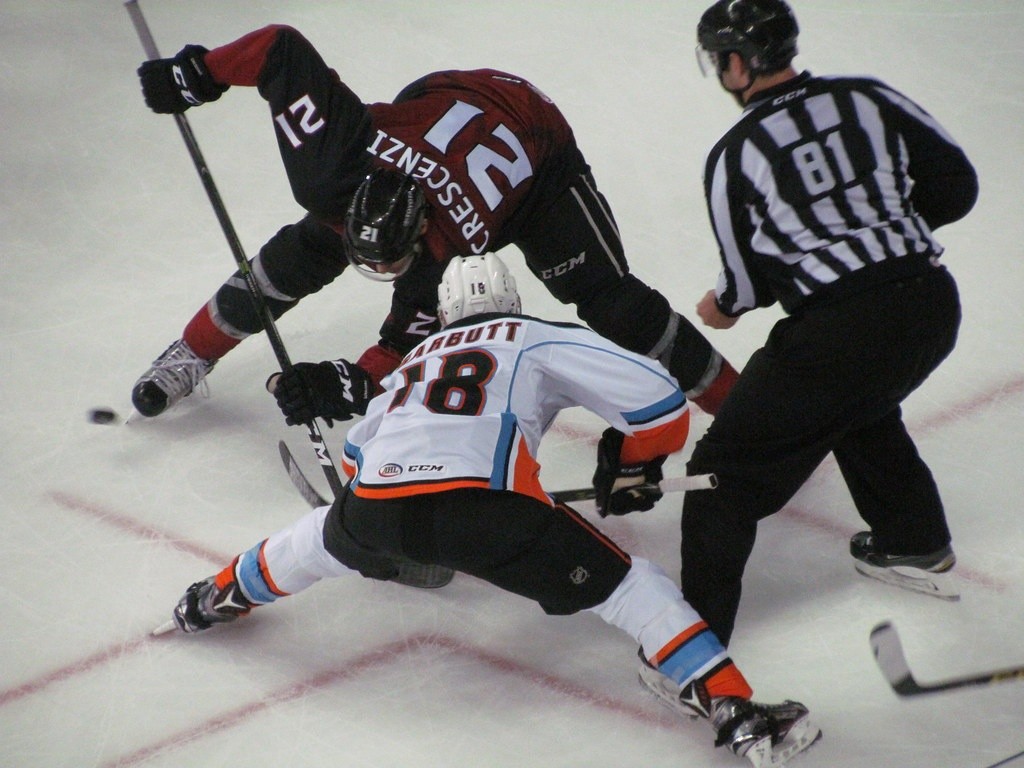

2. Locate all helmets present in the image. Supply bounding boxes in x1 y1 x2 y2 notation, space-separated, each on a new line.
436 251 522 329
696 1 800 78
341 166 430 281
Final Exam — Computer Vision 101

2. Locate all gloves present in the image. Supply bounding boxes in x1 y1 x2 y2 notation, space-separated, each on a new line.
586 428 669 518
136 43 230 114
273 358 374 428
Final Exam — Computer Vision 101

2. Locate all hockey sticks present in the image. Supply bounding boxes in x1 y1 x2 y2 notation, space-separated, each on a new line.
276 435 720 513
123 0 349 504
868 618 1022 699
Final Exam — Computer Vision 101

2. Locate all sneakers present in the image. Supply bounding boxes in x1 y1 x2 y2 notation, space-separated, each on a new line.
705 694 823 767
850 531 963 602
149 574 249 635
123 339 220 425
639 666 700 721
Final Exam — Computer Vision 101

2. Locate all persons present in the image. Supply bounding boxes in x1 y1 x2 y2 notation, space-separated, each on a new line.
629 0 981 723
149 250 821 768
136 22 741 426
